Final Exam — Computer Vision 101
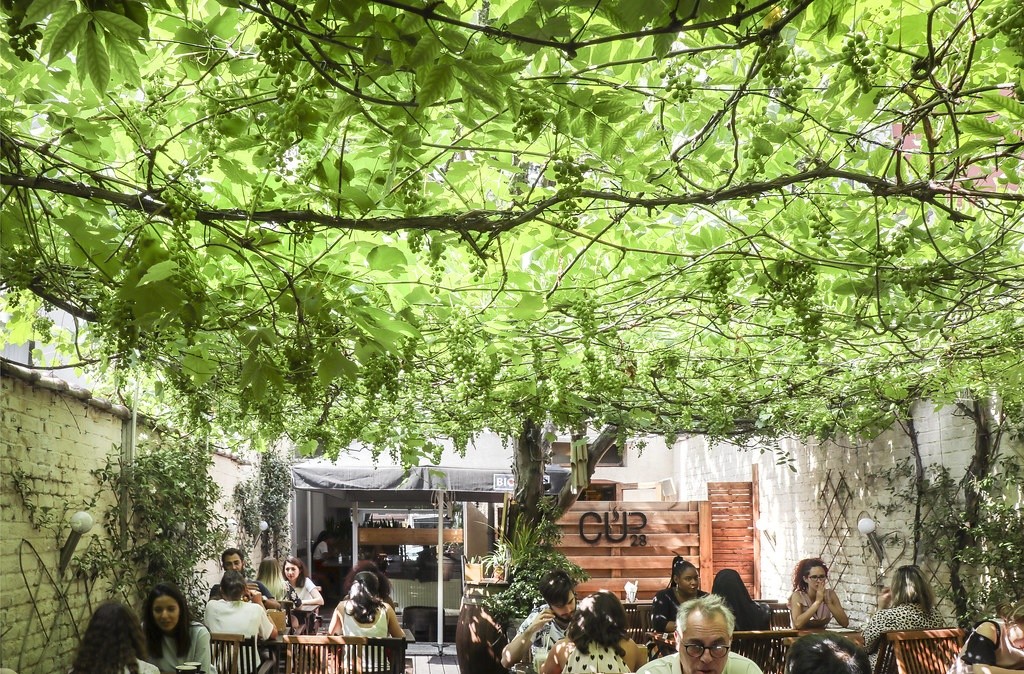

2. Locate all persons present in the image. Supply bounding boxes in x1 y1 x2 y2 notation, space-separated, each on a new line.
712 568 767 670
314 530 341 601
946 598 1024 674
539 588 646 674
783 632 872 674
282 558 325 607
861 565 944 674
634 593 764 674
328 562 406 673
67 602 161 674
500 572 579 669
789 558 850 641
650 557 710 656
257 556 303 630
137 583 211 674
203 549 284 674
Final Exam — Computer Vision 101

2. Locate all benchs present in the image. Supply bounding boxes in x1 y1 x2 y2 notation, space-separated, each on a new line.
387 578 462 616
616 603 970 674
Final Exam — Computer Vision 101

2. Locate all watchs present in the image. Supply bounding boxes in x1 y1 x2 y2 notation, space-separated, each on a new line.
262 595 268 603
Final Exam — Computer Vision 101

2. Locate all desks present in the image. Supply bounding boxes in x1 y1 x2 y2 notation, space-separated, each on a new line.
402 606 445 643
644 627 861 649
619 599 654 604
401 629 416 643
322 560 351 594
291 604 320 634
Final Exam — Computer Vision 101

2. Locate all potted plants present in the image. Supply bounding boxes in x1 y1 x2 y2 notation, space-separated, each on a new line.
482 580 543 663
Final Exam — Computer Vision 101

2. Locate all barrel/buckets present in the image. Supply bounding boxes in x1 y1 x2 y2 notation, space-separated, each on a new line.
455 583 523 674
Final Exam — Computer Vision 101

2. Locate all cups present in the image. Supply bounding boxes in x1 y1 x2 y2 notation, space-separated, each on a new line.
184 661 202 674
176 666 197 674
531 647 550 674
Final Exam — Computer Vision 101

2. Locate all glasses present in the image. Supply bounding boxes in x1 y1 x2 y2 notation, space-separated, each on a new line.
677 631 729 658
807 575 827 582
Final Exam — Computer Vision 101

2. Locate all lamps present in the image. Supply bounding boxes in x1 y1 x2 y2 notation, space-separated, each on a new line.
858 518 883 562
254 521 268 548
61 511 93 576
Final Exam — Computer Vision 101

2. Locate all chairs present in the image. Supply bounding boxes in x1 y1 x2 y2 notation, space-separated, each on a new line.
283 634 408 674
210 586 322 674
